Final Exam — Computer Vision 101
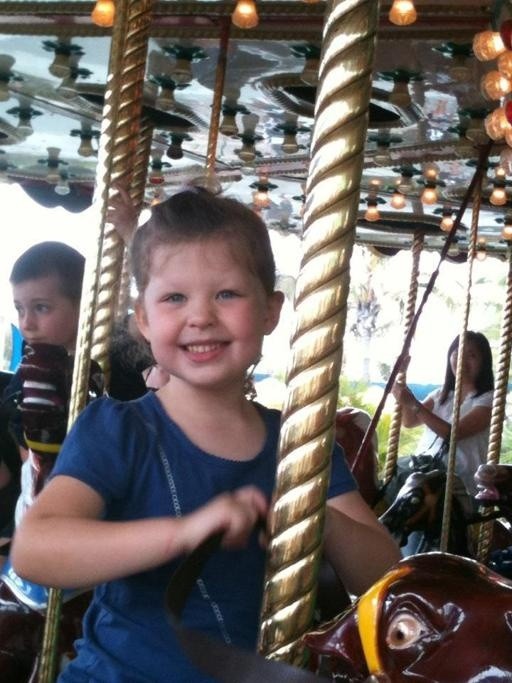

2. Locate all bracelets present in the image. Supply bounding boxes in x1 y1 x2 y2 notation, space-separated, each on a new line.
410 400 421 415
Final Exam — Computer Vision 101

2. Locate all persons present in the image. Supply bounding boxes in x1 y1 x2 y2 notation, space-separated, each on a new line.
0 371 30 683
387 332 496 559
4 241 153 488
7 187 405 683
